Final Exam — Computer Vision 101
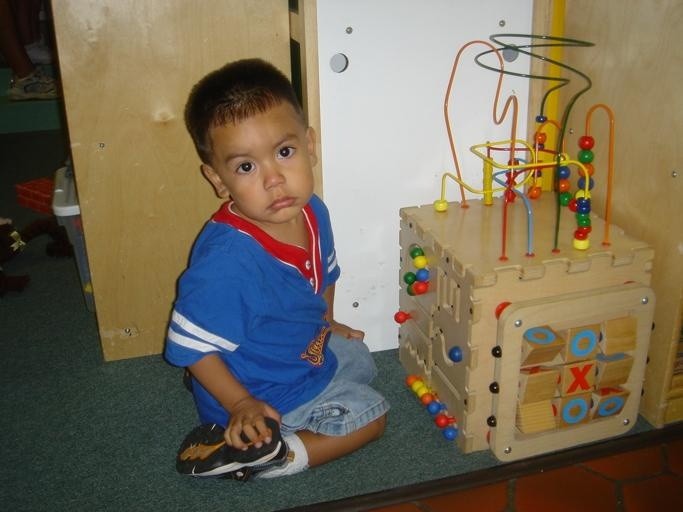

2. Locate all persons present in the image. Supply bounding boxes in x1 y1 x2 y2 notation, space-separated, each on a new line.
163 57 390 481
0 0 63 103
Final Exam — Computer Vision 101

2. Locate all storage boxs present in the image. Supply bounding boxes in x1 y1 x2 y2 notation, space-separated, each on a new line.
52 167 98 315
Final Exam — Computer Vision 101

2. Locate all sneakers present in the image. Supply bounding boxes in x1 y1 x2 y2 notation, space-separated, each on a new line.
175 421 282 479
